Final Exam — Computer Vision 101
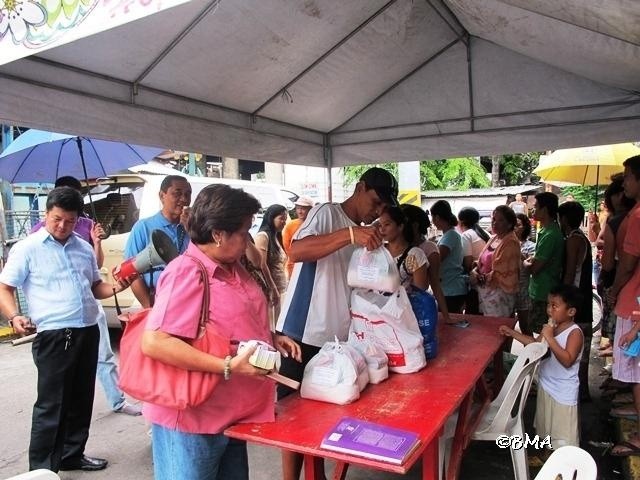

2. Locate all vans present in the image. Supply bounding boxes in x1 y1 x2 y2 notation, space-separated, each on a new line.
81 173 300 329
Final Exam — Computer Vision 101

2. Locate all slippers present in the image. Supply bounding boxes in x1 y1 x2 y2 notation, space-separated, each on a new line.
597 342 640 457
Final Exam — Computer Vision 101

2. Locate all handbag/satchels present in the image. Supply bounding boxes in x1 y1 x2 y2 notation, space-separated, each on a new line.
117 307 231 411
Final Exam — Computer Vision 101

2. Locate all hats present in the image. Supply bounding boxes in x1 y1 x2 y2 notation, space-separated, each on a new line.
359 167 400 208
294 197 313 207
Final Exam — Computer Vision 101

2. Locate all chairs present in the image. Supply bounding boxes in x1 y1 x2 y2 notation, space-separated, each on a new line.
438 343 549 480
531 446 598 480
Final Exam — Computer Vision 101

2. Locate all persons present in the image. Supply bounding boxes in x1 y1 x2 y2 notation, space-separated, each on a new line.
0 154 640 478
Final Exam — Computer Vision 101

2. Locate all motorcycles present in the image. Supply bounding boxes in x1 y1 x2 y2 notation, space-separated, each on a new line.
592 284 601 335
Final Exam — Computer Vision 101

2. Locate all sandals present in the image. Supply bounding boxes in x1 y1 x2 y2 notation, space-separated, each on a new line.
529 455 544 466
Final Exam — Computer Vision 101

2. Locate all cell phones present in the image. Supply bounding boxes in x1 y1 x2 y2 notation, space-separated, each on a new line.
22 323 36 330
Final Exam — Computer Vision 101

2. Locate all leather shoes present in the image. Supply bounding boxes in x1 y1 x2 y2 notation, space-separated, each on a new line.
60 455 108 471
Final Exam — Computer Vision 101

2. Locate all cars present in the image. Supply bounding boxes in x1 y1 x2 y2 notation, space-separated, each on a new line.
477 210 494 232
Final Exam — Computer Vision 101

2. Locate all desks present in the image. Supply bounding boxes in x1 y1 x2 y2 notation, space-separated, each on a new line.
223 312 519 480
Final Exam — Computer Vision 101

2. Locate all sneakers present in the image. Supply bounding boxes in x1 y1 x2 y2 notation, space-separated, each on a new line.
114 403 142 415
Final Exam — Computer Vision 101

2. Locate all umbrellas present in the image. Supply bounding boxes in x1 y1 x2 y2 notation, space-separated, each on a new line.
532 142 640 222
0 129 166 239
